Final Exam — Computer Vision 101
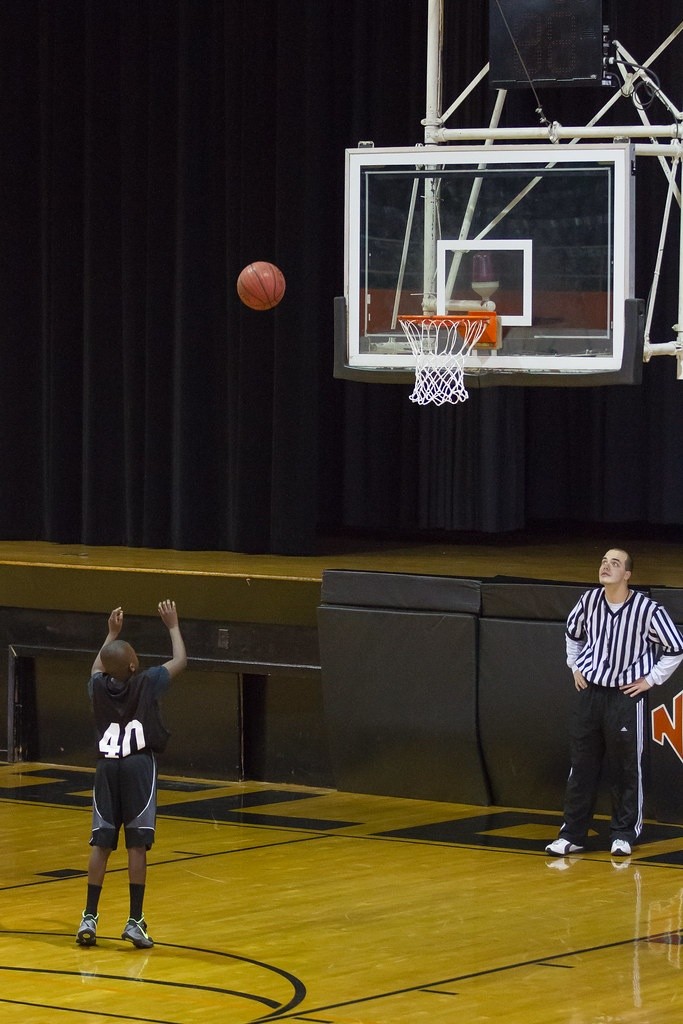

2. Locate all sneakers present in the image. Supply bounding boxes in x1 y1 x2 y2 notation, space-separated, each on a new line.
76 910 99 944
545 838 584 856
611 837 631 855
123 915 154 949
544 853 582 870
611 856 630 870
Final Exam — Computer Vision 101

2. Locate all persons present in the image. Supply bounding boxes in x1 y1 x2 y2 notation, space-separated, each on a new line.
73 599 187 948
545 548 683 856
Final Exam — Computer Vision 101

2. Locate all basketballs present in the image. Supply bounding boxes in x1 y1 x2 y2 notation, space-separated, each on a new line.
236 261 286 310
